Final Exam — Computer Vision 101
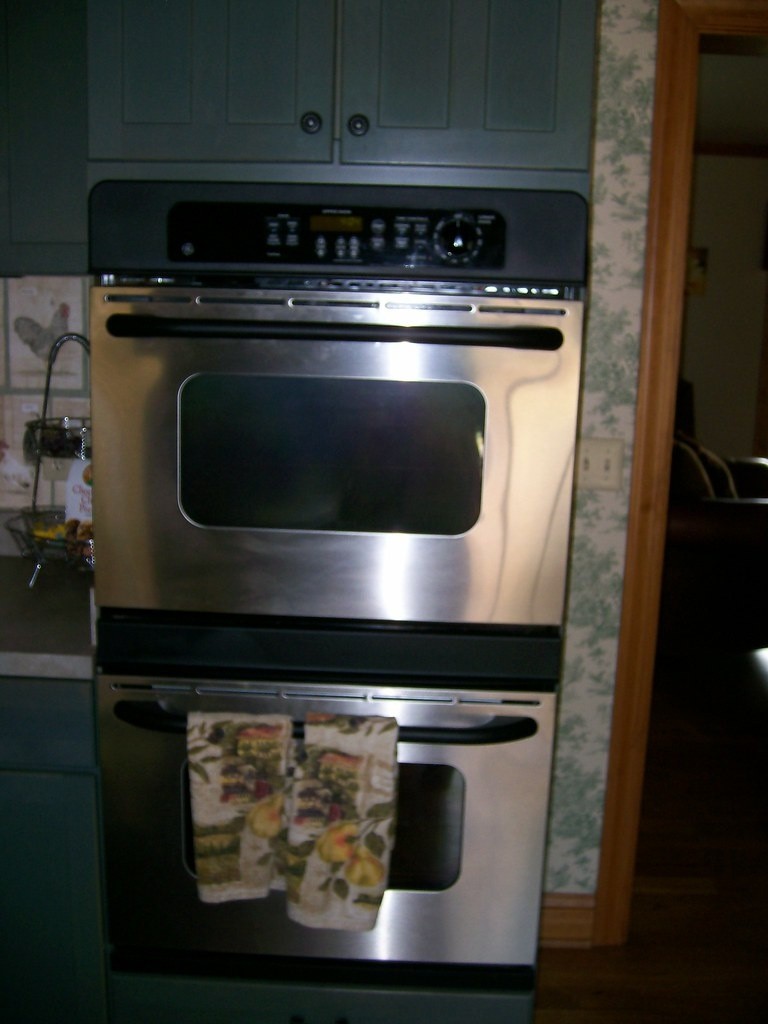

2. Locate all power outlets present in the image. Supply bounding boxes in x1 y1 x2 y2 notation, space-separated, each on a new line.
573 436 625 493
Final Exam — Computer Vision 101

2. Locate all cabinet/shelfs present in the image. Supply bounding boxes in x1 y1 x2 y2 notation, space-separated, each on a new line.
0 675 108 1024
87 2 600 200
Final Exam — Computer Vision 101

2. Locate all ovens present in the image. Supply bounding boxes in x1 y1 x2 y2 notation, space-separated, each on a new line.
86 174 592 993
